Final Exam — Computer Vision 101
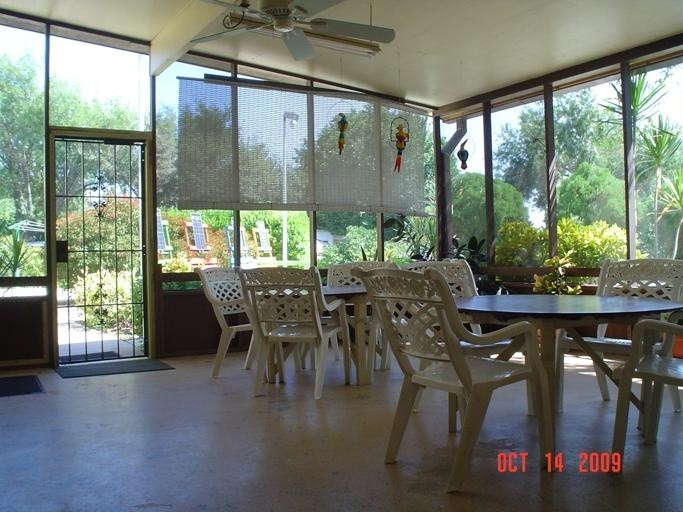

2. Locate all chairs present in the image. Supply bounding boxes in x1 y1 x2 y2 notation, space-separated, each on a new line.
608 310 683 477
193 265 312 385
324 260 393 287
231 264 351 401
447 293 683 429
368 259 484 414
555 257 682 417
347 264 554 494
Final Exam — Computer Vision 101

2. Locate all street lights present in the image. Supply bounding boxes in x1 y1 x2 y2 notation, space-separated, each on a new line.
282 112 299 269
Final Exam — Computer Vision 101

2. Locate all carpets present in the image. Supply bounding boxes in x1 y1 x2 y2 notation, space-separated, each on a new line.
0 374 47 399
51 356 176 381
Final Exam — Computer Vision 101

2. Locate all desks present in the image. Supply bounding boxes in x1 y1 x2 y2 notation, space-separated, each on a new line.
320 285 370 388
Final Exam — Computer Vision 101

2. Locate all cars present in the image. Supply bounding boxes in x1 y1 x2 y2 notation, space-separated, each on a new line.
23 233 46 249
316 229 333 262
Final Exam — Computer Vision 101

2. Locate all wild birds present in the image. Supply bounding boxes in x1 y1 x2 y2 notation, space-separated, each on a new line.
457 139 469 169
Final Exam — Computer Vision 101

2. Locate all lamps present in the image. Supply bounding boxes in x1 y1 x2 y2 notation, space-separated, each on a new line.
226 1 384 61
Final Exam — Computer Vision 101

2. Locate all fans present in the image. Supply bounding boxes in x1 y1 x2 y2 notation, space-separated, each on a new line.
188 0 399 64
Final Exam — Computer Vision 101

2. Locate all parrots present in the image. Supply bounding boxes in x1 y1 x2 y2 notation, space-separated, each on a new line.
393 124 406 172
338 112 348 155
